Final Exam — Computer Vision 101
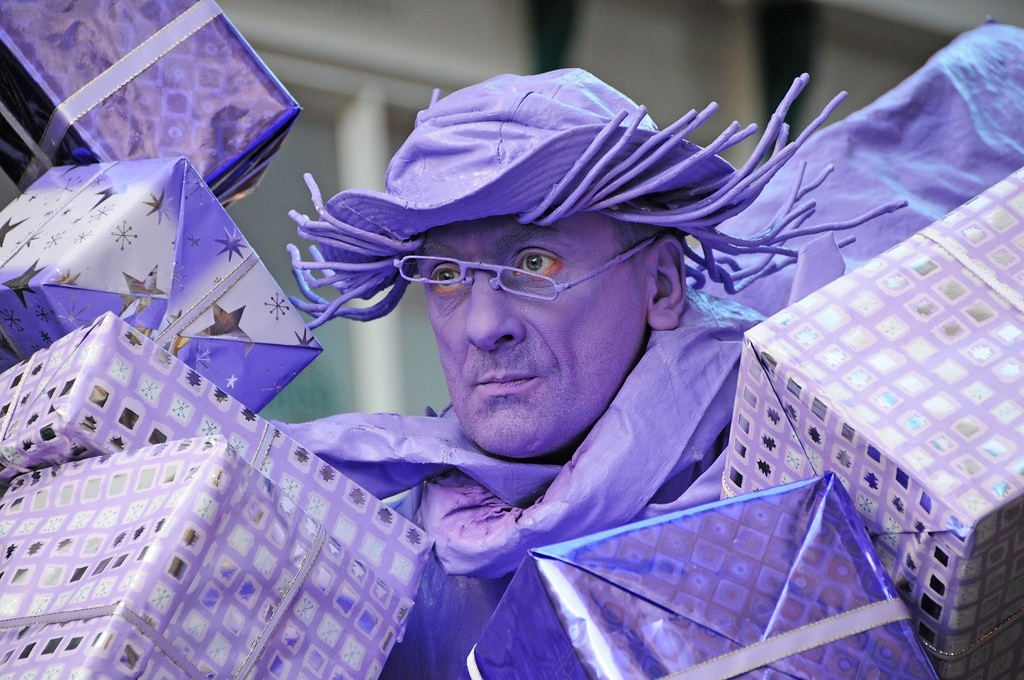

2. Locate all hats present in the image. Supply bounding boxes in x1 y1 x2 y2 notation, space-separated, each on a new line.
319 66 736 297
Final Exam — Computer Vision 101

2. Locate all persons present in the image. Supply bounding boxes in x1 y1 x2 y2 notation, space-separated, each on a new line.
122 66 960 680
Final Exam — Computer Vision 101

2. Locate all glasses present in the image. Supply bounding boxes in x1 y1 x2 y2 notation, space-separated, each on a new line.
392 234 664 303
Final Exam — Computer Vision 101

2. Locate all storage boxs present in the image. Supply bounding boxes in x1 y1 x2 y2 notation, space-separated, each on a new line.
0 1 1024 680
